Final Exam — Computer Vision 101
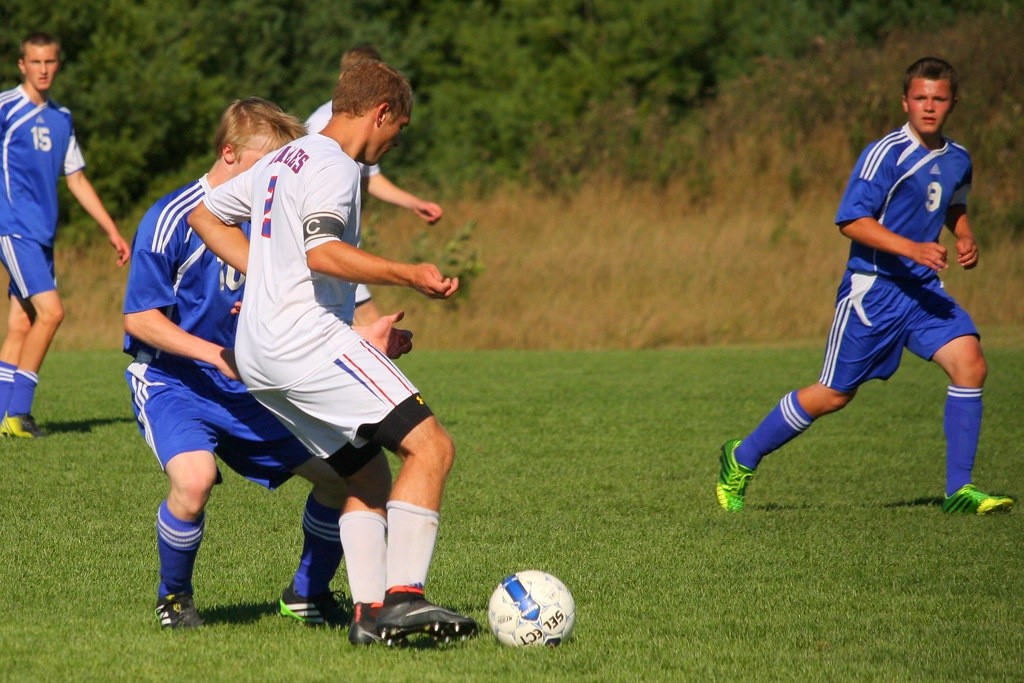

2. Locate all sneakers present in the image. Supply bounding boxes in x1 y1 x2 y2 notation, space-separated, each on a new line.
944 485 1014 515
375 597 478 647
2 410 47 440
349 602 409 646
279 580 350 626
155 592 203 629
715 439 755 511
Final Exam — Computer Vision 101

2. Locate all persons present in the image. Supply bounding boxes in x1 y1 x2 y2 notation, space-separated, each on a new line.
301 45 446 326
188 55 492 646
1 32 130 437
714 57 1016 516
122 98 352 631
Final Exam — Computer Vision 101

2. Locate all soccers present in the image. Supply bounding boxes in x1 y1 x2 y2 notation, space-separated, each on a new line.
487 570 576 649
